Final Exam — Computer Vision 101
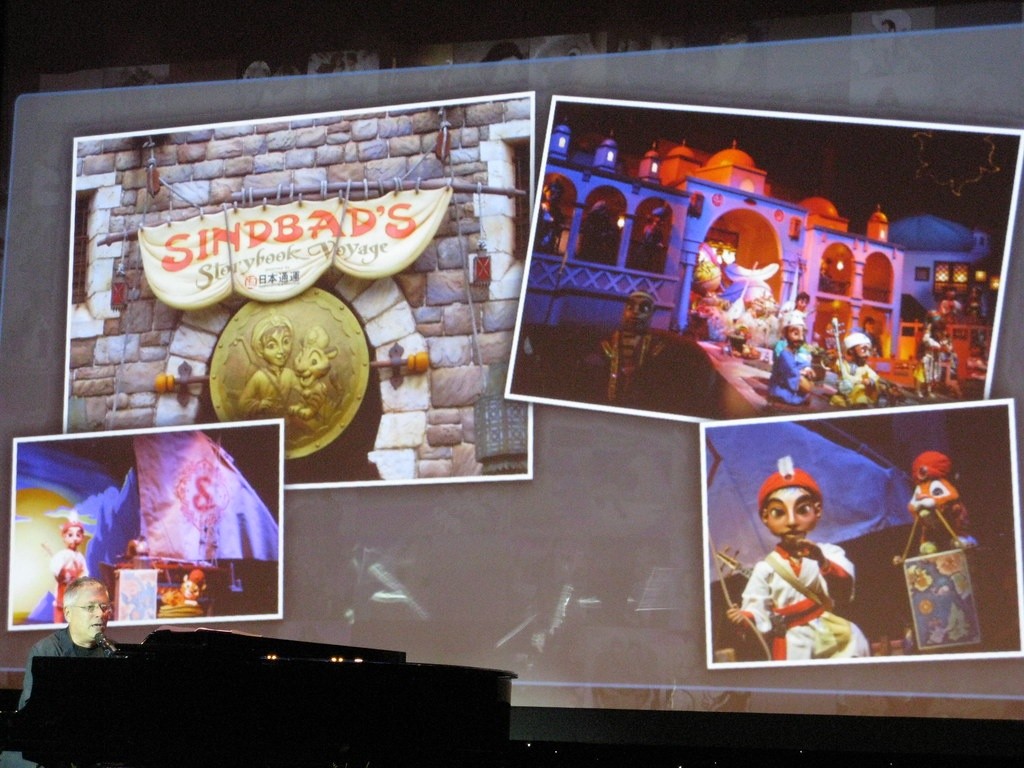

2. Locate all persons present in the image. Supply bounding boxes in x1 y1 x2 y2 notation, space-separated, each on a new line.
20 579 120 710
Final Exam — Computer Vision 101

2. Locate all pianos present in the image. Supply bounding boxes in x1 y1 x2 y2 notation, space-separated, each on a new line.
8 628 520 768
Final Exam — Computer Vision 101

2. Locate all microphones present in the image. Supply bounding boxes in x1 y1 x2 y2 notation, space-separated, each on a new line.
93 633 119 654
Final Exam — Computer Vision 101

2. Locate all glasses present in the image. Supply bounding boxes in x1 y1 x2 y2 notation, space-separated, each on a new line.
74 602 113 615
